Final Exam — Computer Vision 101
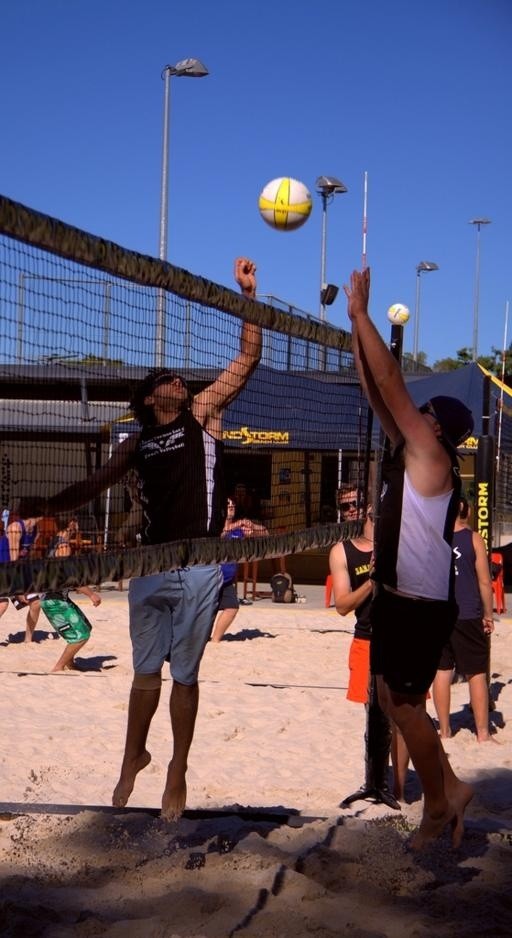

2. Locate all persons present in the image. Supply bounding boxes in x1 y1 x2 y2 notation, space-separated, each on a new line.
341 266 475 852
16 256 258 825
209 497 268 643
1 467 143 673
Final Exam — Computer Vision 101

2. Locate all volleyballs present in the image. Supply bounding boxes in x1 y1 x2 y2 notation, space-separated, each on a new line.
259 176 312 231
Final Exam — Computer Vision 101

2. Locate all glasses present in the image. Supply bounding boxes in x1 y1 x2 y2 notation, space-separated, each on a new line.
339 499 364 511
418 402 436 418
153 375 187 389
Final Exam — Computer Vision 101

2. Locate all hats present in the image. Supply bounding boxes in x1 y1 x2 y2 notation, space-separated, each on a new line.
429 395 474 461
